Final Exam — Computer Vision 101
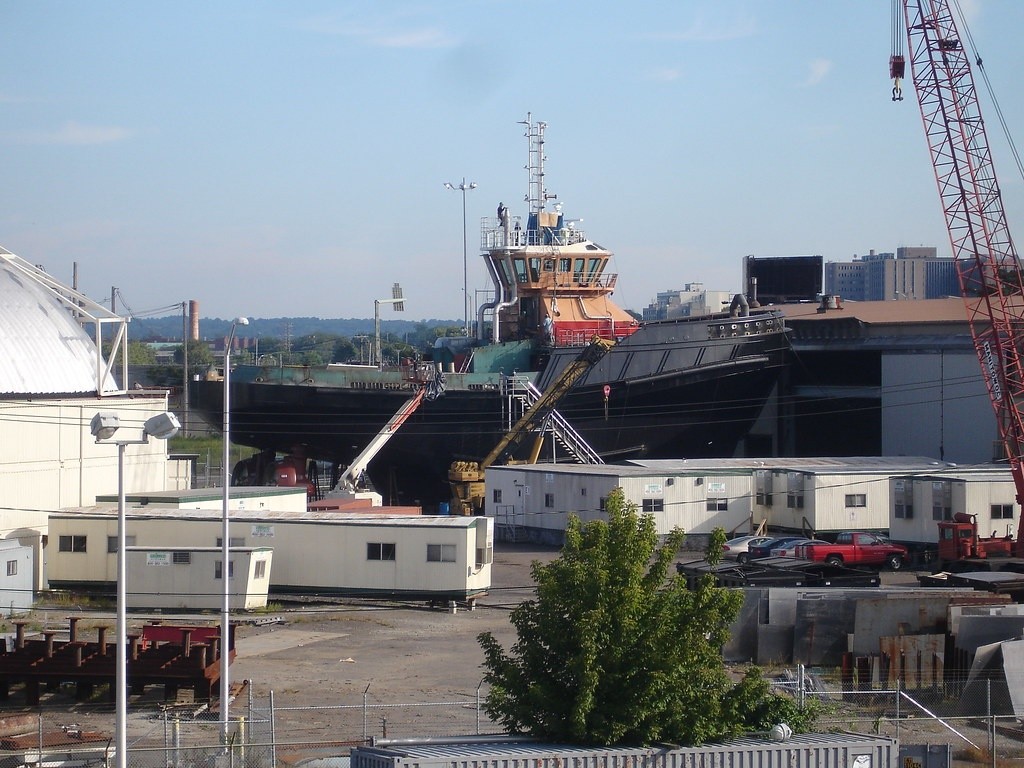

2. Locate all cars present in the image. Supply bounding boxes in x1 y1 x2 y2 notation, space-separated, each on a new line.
749 536 810 558
718 535 775 565
769 539 835 559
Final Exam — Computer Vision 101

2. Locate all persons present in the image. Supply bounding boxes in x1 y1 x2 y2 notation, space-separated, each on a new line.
993 531 997 538
497 202 505 226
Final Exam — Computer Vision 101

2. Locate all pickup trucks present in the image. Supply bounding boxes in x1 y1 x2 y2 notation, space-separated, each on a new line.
795 531 912 572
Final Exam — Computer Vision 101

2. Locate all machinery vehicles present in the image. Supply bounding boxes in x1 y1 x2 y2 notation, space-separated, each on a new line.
885 1 1024 566
313 361 454 520
441 337 618 516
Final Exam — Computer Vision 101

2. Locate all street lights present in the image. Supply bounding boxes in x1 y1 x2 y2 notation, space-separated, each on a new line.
220 318 266 755
89 411 182 768
444 176 479 334
